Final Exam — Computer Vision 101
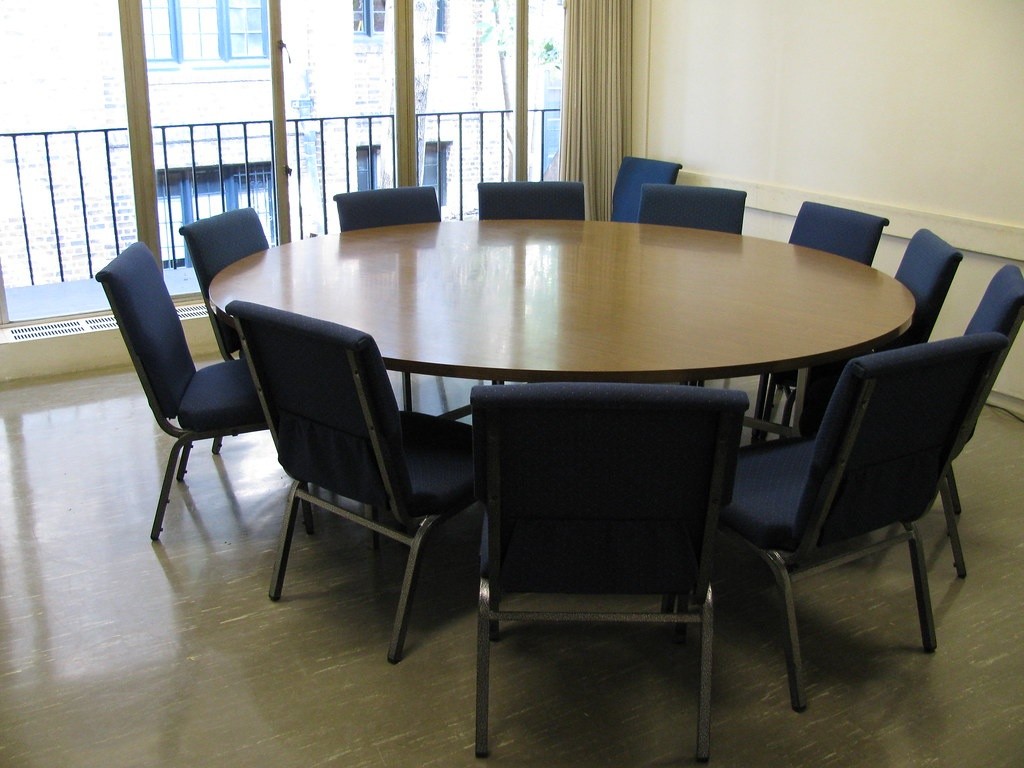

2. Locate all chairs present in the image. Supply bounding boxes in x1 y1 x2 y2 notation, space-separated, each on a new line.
96 156 1024 763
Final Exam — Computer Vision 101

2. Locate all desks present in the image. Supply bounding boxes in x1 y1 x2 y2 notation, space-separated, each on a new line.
208 219 915 418
